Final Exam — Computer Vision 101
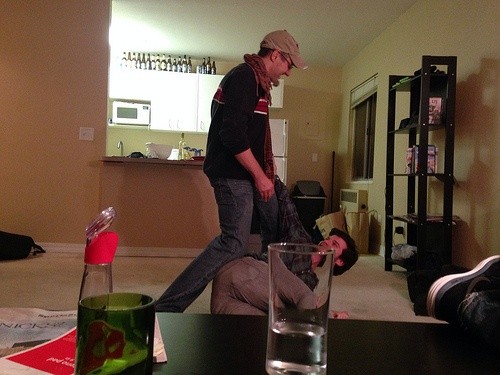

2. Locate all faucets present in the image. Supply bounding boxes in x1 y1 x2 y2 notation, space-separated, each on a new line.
118 141 123 156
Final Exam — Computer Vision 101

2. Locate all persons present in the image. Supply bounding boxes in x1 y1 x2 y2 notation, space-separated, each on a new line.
158 30 307 313
210 163 357 318
428 254 500 354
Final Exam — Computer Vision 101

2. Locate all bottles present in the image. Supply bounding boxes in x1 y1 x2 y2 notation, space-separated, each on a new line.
178 132 186 160
78 209 119 302
122 52 217 75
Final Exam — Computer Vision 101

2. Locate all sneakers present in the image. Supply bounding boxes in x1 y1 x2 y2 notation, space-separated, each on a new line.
428 254 500 322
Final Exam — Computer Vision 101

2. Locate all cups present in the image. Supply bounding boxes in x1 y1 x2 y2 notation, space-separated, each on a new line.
74 292 157 375
265 243 336 374
183 147 194 160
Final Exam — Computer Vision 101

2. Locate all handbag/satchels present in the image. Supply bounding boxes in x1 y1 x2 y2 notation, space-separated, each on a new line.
315 211 347 240
345 209 377 254
0 231 44 262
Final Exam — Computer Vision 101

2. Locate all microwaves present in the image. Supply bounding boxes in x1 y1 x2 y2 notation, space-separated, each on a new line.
112 101 150 125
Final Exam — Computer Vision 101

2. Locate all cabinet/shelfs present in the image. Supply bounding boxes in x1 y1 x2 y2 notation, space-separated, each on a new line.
108 64 285 133
384 54 457 271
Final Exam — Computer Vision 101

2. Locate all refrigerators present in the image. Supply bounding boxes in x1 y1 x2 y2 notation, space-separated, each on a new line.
269 119 288 187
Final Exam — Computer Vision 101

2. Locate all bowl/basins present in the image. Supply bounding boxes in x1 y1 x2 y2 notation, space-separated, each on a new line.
145 142 173 160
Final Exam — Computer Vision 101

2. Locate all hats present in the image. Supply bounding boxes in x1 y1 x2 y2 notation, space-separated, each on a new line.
261 29 306 69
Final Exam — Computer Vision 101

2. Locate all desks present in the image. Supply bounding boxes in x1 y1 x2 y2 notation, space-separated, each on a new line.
0 314 500 375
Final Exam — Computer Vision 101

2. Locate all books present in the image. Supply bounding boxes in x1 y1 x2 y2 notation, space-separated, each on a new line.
406 97 442 173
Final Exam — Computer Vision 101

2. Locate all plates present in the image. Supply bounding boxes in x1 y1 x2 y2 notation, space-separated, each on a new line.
192 156 205 160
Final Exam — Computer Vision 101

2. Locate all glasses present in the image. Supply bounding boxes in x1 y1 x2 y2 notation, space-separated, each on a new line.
278 50 293 70
